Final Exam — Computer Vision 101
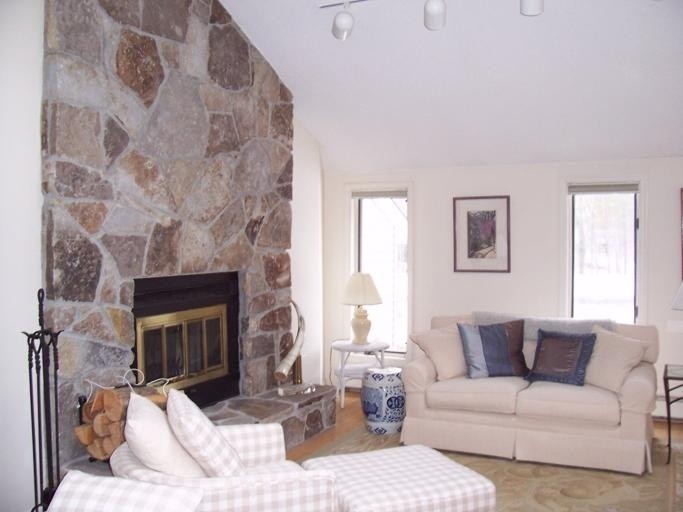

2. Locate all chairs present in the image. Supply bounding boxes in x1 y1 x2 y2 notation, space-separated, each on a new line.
107 423 340 512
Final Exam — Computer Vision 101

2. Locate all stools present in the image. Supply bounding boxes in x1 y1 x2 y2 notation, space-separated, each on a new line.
301 445 496 512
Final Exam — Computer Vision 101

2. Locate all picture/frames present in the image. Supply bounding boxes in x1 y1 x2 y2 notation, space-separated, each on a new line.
453 195 511 273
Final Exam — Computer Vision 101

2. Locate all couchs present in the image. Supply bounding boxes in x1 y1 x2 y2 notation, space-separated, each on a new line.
398 312 658 476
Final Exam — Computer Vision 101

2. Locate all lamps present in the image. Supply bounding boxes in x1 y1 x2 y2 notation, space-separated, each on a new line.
319 0 543 41
340 272 382 346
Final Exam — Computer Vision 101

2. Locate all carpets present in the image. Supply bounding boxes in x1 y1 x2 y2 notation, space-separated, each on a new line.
296 436 683 512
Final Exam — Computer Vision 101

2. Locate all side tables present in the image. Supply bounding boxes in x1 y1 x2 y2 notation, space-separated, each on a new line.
332 339 389 408
664 364 683 464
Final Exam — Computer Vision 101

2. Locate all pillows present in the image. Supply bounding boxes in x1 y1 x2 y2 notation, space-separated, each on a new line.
44 470 202 512
455 320 530 379
165 389 244 478
520 328 595 386
585 321 653 393
408 323 468 380
124 390 200 476
